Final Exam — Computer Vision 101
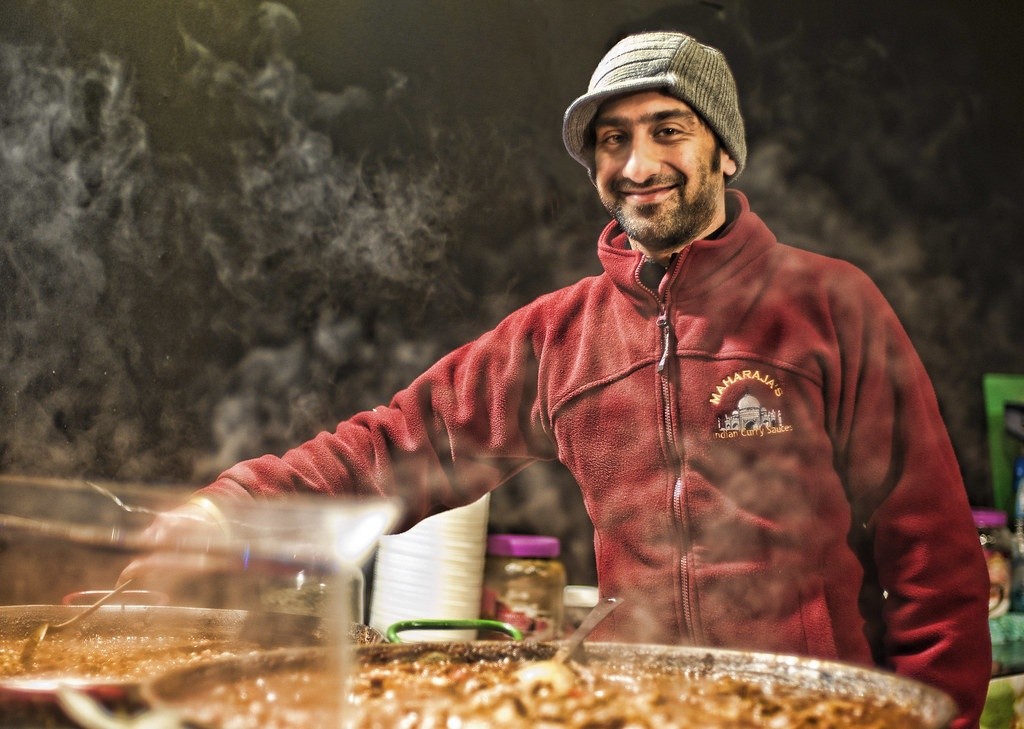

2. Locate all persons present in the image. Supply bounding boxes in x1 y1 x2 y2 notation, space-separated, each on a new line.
115 33 992 729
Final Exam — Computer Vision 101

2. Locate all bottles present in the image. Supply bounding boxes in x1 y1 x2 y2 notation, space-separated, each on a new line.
968 507 1016 623
475 533 568 642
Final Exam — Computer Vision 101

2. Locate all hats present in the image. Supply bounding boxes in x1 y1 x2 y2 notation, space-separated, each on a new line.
562 32 747 187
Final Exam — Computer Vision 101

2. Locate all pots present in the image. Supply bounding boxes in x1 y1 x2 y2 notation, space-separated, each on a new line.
0 590 959 729
